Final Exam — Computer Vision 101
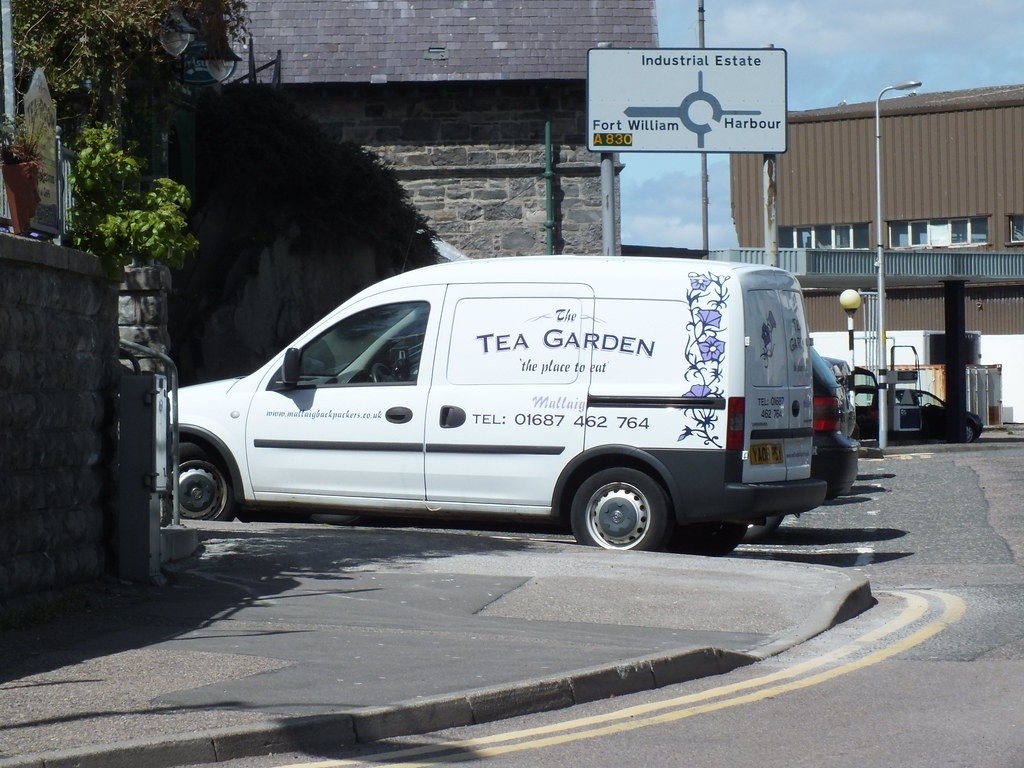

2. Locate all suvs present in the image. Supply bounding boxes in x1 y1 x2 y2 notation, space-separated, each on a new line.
741 340 859 545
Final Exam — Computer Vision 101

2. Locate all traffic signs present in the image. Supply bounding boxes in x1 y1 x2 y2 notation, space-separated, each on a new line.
584 45 789 157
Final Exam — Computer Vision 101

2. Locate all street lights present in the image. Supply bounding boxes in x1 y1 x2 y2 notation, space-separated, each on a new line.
875 80 925 451
839 288 861 410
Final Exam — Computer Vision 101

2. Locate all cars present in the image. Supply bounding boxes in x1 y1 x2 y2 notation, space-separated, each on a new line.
850 387 982 447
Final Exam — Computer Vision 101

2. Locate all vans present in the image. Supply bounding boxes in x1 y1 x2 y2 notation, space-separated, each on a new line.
170 253 827 559
